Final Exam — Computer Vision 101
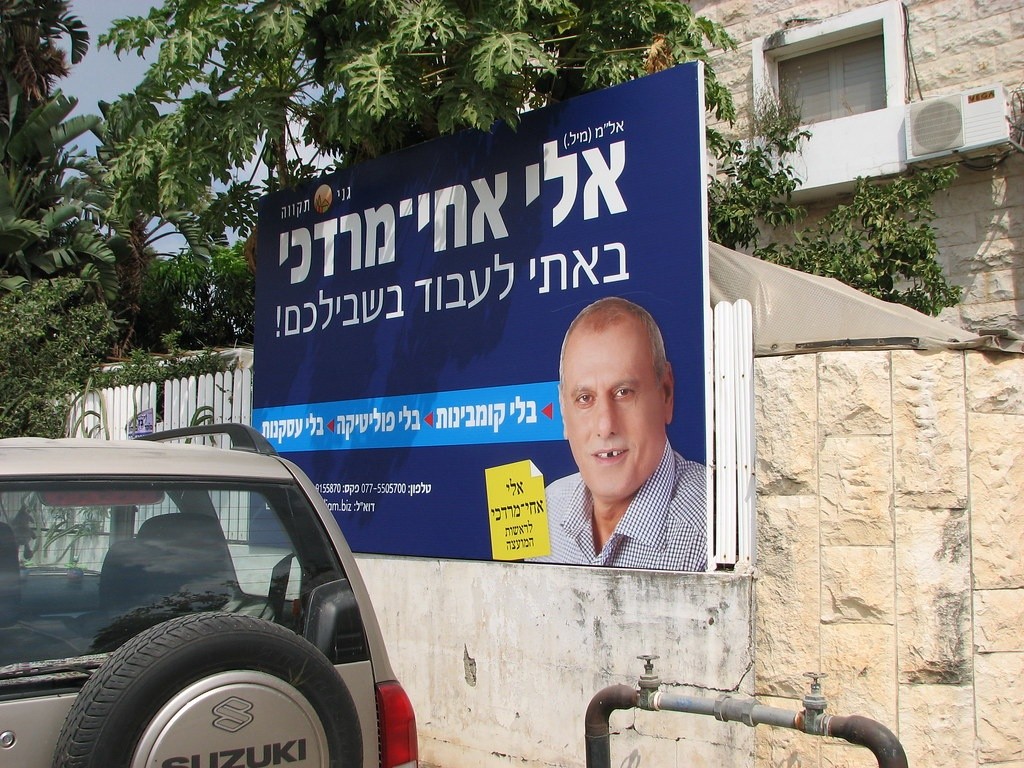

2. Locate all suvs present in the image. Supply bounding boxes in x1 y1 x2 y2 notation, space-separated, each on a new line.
0 420 415 768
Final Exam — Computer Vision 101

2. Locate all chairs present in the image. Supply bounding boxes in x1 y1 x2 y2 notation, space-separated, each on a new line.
97 513 225 610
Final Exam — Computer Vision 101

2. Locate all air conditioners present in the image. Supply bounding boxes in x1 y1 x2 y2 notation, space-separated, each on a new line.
904 84 1012 170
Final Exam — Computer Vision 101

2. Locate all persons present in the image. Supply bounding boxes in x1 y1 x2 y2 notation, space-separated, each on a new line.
525 296 707 574
